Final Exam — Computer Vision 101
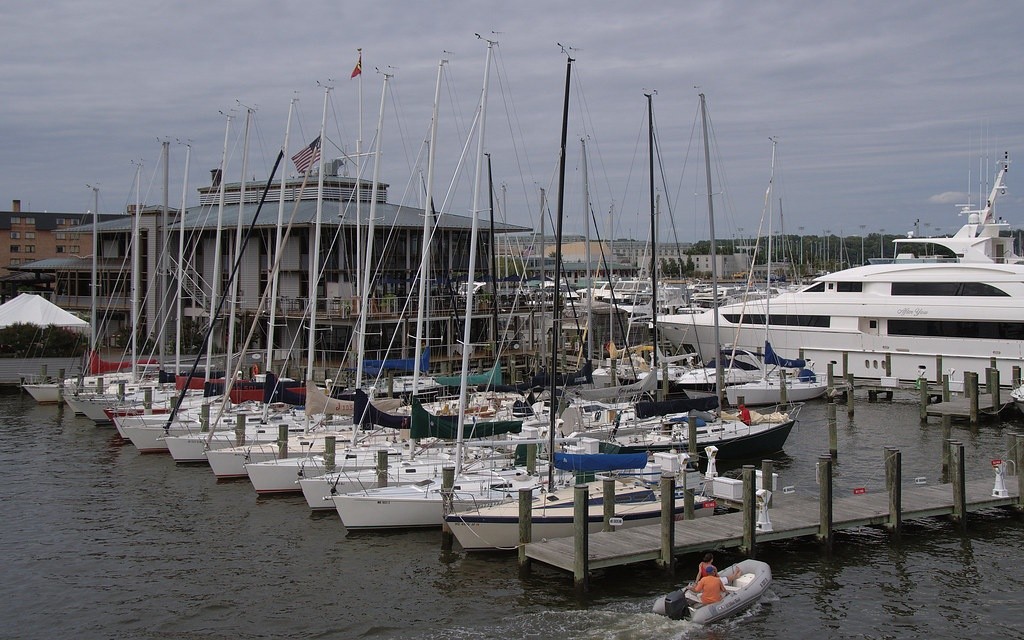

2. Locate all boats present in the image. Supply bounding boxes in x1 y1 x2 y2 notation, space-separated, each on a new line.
651 558 772 627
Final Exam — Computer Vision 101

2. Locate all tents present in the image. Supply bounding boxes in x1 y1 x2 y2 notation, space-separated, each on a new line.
0 291 92 329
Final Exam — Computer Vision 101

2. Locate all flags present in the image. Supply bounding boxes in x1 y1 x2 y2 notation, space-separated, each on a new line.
291 135 322 173
351 52 361 79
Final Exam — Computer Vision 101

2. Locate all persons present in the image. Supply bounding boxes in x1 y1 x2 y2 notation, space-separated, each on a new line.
687 567 726 606
692 552 741 587
736 403 752 426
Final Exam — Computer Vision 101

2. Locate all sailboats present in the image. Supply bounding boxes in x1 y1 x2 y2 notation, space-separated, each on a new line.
13 29 1024 551
